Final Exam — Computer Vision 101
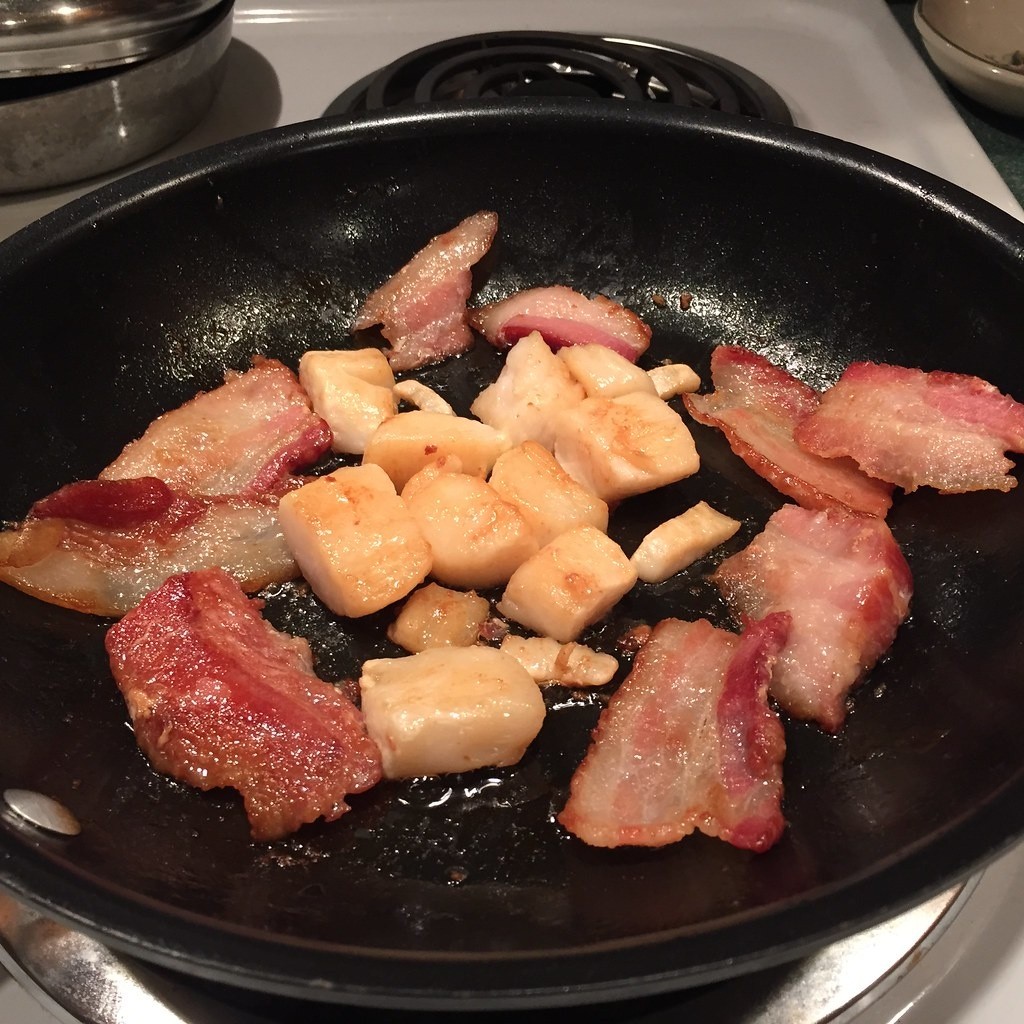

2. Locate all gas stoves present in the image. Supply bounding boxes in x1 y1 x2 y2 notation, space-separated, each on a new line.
0 1 1024 1024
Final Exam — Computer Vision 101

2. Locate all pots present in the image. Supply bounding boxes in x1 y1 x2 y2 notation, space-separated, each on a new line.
912 0 1024 118
0 0 236 197
0 29 1024 1017
